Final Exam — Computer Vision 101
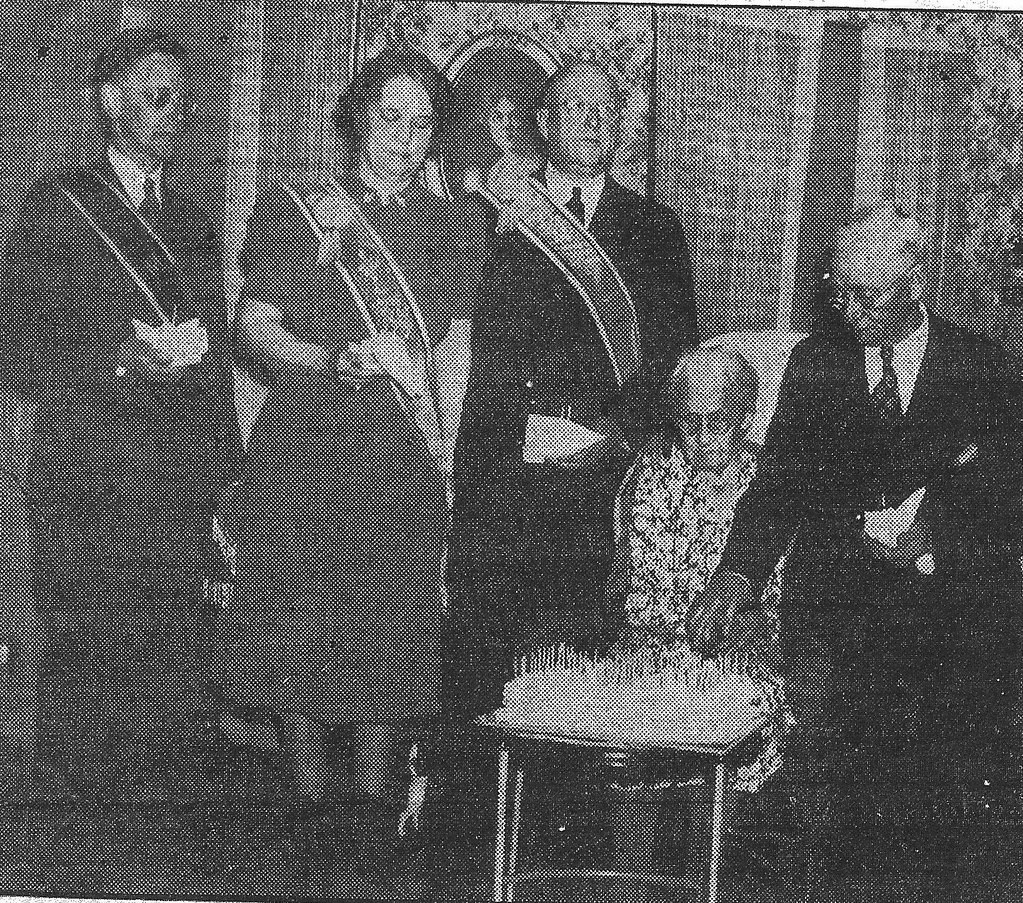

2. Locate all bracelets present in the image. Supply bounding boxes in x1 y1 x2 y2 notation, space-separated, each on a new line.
328 345 351 391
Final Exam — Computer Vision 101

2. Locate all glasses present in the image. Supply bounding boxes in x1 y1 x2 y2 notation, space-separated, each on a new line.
119 86 190 116
831 263 919 310
679 411 733 432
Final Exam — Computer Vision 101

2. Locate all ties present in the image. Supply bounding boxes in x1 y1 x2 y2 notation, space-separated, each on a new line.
566 186 584 226
869 345 902 432
138 178 160 228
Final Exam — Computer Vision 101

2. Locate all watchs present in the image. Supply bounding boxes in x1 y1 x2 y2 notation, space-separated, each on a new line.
914 553 936 575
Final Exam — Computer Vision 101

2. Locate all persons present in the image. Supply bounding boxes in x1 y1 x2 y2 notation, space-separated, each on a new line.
208 45 482 875
684 206 1022 903
5 28 244 805
608 347 797 903
483 82 548 178
429 62 699 903
